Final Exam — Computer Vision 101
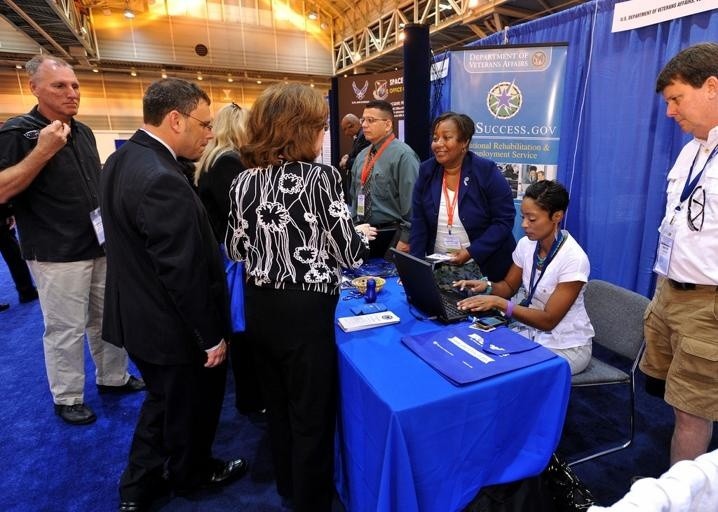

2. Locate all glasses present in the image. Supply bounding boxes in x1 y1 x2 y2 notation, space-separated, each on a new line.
687 186 705 232
359 117 387 124
22 119 64 140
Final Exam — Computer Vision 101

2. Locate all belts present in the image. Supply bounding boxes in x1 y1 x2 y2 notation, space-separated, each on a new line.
671 280 695 289
371 219 400 229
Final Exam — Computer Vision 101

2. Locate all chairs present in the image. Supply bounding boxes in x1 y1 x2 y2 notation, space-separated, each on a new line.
567 279 651 467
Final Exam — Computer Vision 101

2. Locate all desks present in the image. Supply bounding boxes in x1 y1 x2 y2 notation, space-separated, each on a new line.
334 257 571 512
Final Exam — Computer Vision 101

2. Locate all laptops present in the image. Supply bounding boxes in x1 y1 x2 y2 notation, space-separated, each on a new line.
388 245 494 324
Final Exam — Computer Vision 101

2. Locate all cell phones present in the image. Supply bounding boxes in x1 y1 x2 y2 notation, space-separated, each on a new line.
476 314 508 330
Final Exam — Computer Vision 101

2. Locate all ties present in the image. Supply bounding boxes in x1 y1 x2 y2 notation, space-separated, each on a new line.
359 147 376 223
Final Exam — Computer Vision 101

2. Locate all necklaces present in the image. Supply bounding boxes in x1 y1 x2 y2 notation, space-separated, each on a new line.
534 233 564 271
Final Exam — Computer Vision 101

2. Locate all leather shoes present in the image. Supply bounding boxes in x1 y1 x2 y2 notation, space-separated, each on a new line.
183 457 249 500
0 303 9 312
96 375 147 393
19 288 38 303
54 402 97 425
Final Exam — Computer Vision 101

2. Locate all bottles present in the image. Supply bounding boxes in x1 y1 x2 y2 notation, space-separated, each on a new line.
364 279 376 303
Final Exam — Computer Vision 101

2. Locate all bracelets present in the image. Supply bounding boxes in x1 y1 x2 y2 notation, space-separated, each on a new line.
506 301 515 319
480 277 493 295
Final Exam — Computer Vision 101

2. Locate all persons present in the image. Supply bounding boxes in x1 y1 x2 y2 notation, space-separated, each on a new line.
0 55 146 425
534 171 545 182
524 166 537 183
349 99 420 256
636 41 718 468
195 102 270 422
0 204 40 312
225 82 378 512
338 113 372 206
99 78 244 512
397 111 517 287
451 179 596 375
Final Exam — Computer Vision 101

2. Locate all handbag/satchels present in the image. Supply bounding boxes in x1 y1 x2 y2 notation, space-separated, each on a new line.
219 244 246 332
401 317 558 387
